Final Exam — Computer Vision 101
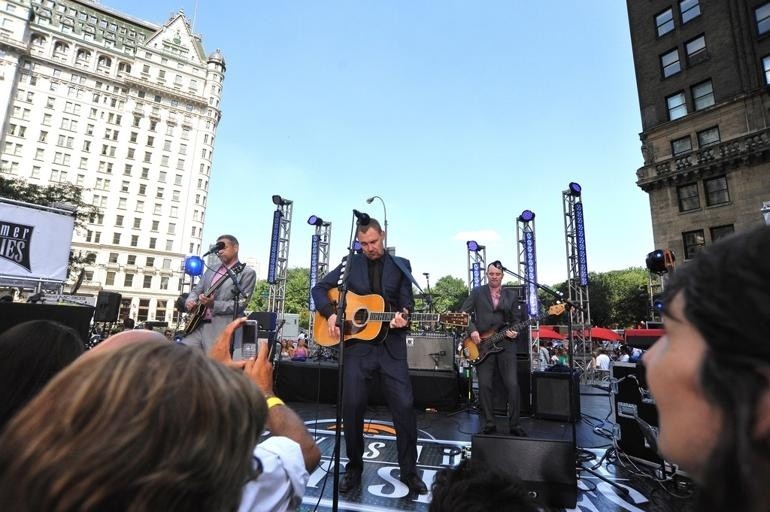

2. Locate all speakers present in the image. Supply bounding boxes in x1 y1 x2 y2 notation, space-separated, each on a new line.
278 359 340 405
0 301 95 344
407 370 461 411
470 433 578 510
94 291 122 322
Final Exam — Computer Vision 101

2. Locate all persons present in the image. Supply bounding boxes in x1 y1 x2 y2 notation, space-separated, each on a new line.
458 262 529 437
311 218 428 494
1 320 85 430
1 341 267 512
93 316 321 512
279 333 311 361
532 298 646 371
123 318 134 332
164 329 172 340
180 235 256 355
640 220 770 511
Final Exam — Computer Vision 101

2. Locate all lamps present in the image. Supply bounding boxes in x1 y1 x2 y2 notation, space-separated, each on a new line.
569 182 581 197
646 249 676 276
307 215 322 226
184 256 203 276
651 293 663 310
519 210 535 221
272 195 283 205
467 241 480 251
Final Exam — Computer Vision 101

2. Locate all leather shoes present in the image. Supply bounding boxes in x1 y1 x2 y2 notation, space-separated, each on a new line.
339 465 363 493
399 470 428 495
482 425 497 433
509 426 530 438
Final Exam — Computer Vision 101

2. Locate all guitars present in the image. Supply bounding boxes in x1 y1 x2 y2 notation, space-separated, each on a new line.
464 304 566 365
184 262 245 334
314 289 470 350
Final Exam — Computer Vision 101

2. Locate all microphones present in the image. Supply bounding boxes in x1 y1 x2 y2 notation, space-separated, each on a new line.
353 209 370 226
494 260 502 270
203 241 225 258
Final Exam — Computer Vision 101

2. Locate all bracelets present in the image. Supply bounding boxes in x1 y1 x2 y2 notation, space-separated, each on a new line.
267 397 284 409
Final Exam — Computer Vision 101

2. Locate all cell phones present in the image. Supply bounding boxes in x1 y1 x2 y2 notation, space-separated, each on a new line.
239 320 258 362
